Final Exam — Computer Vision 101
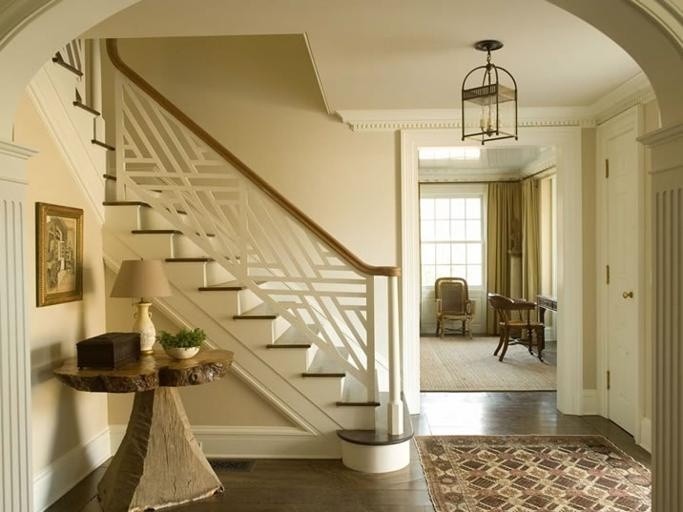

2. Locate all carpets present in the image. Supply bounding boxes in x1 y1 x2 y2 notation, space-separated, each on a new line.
419 333 557 393
412 431 653 512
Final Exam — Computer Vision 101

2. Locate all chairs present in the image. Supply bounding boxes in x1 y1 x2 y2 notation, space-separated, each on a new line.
486 292 545 363
432 277 476 337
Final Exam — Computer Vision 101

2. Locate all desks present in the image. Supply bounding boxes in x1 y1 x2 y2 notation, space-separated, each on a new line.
51 351 235 511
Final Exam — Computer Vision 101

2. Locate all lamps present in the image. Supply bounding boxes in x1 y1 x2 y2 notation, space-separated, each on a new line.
458 41 520 144
111 255 170 356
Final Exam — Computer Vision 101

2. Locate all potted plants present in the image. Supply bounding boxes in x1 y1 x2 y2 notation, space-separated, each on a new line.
154 328 205 361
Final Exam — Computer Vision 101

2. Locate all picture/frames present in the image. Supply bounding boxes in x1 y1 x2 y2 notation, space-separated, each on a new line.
34 201 83 307
